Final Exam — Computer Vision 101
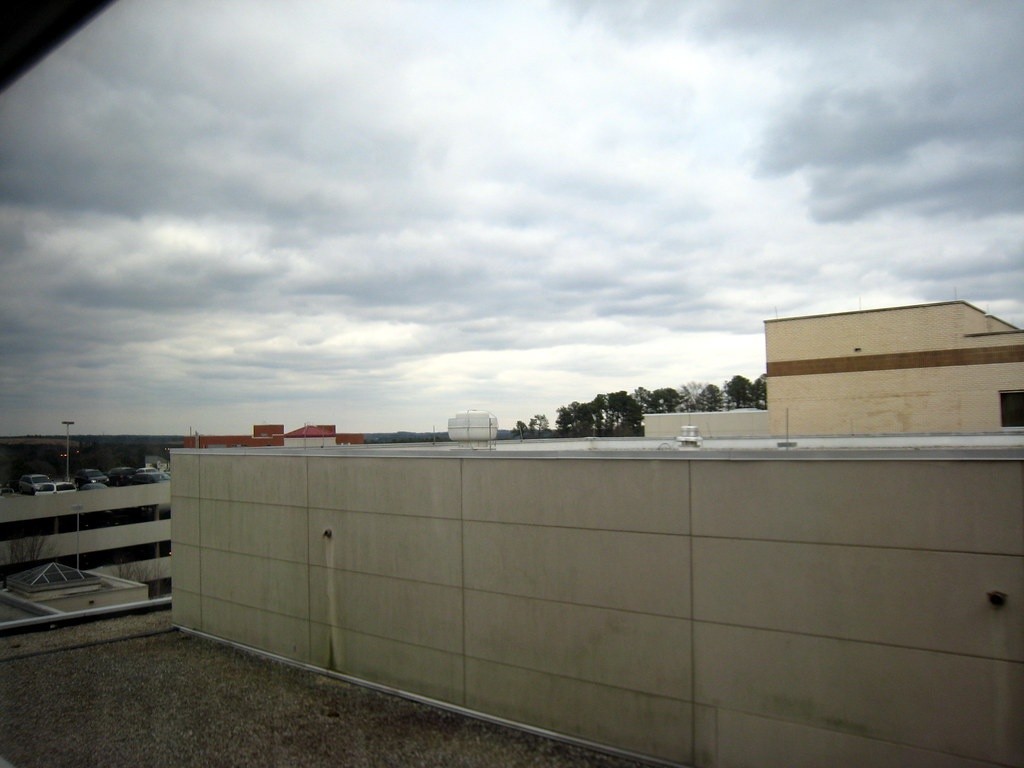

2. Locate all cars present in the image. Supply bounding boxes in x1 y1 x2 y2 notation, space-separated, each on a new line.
0 480 19 496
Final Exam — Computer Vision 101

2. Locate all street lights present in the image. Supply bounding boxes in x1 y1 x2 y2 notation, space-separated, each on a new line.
61 420 75 483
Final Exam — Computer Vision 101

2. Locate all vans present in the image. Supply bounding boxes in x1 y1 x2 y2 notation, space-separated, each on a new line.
107 467 138 486
34 482 77 494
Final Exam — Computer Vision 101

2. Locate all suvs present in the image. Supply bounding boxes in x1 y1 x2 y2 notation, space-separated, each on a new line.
137 466 164 482
75 467 111 486
18 474 53 496
